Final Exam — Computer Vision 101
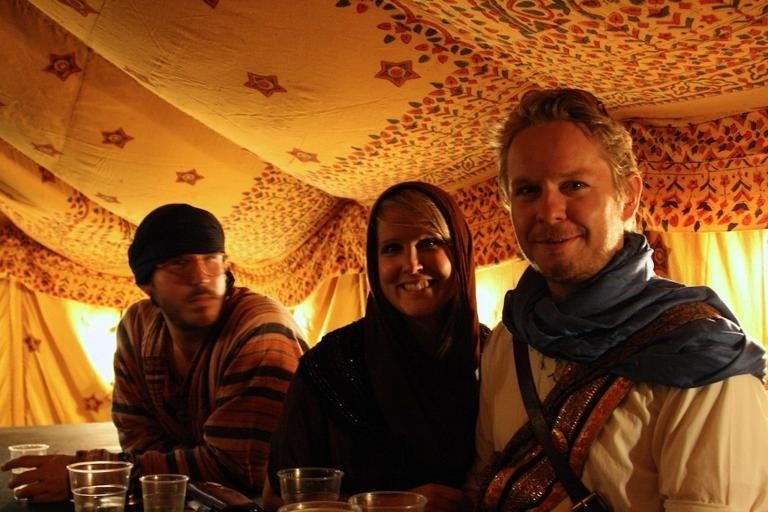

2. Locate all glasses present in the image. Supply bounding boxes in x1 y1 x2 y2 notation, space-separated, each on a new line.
153 256 227 275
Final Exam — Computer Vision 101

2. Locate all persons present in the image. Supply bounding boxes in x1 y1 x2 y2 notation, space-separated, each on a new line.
473 86 767 512
1 204 307 507
266 181 491 512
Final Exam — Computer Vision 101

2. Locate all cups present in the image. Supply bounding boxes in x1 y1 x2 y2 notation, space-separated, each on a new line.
277 468 343 505
9 442 49 501
140 473 188 512
66 457 132 512
348 491 428 511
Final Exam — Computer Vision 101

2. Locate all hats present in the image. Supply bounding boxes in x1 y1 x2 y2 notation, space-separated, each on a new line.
128 203 225 283
